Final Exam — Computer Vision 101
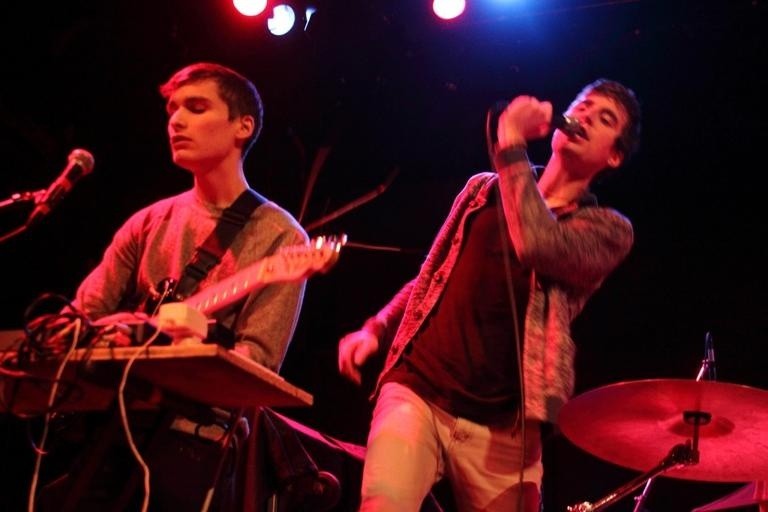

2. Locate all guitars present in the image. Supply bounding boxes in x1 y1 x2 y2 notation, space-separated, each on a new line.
132 234 341 345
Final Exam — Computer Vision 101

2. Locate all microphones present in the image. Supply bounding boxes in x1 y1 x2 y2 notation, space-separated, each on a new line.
33 149 97 217
498 101 581 131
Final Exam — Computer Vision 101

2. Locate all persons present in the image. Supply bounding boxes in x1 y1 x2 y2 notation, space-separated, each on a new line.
39 62 310 512
334 78 641 512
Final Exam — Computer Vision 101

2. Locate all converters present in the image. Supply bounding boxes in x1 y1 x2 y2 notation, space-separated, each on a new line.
158 302 208 339
202 319 236 349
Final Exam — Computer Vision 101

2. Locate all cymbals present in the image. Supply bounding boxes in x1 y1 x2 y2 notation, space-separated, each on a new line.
557 379 768 483
